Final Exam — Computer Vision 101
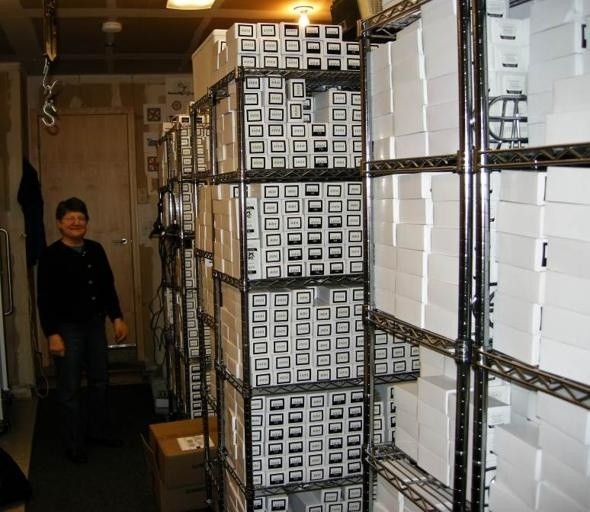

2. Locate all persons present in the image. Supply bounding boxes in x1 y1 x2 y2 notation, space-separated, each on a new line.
37 197 128 465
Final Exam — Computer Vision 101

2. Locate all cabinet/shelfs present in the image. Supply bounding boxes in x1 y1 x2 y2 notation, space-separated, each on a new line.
471 0 590 512
356 0 471 512
149 65 420 512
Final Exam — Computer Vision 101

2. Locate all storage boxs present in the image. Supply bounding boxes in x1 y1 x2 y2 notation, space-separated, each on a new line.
140 434 211 512
191 29 229 102
149 416 218 489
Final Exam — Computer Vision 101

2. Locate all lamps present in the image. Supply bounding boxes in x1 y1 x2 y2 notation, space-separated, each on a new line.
160 0 221 11
294 5 313 28
